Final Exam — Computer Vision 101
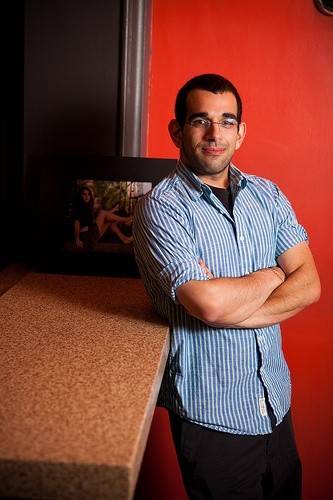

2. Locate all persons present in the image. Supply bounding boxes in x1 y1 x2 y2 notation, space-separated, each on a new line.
72 186 133 249
131 73 321 500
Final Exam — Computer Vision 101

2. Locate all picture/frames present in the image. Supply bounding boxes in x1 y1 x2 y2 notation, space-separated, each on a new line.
40 155 177 279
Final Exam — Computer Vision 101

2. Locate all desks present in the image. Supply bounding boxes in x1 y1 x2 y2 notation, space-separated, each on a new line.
0 272 172 500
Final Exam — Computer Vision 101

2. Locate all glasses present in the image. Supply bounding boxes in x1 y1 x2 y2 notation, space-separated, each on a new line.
184 116 240 129
